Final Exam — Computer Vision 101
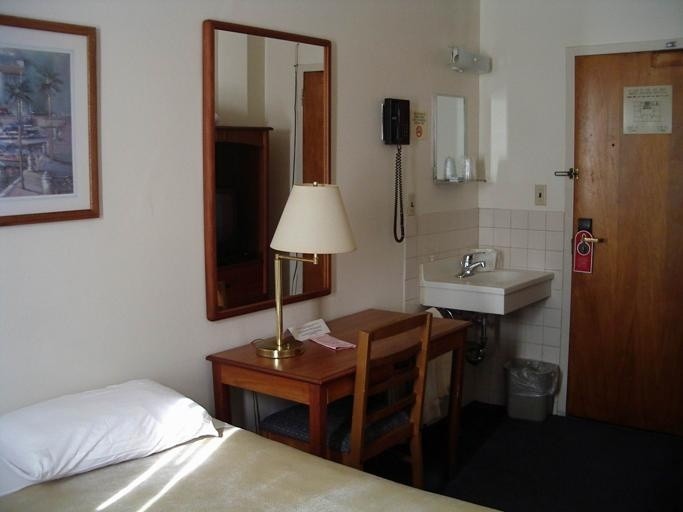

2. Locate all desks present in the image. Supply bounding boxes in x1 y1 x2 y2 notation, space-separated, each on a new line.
206 308 475 448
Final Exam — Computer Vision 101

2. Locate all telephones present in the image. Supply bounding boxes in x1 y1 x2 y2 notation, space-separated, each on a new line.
383 98 411 145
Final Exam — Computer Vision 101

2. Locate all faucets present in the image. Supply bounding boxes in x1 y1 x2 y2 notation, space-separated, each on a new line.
457 254 486 278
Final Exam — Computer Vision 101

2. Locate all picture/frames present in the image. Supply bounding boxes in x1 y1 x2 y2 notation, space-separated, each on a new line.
0 13 100 227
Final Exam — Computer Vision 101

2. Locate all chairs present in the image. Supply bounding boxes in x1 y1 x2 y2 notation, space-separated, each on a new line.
260 312 432 489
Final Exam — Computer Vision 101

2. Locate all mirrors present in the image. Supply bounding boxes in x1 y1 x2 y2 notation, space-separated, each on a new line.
432 93 466 183
202 19 332 322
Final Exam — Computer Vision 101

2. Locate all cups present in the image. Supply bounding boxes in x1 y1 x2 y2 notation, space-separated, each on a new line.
444 156 456 181
462 158 477 181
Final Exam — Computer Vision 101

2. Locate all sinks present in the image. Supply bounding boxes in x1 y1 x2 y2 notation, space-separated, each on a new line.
419 250 554 316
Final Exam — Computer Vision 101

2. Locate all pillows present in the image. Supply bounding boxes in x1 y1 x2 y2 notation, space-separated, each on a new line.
0 378 220 484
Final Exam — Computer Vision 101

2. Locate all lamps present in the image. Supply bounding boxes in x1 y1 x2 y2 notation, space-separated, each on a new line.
255 182 358 360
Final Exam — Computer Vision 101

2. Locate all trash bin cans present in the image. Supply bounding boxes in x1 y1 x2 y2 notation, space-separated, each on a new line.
508 359 560 421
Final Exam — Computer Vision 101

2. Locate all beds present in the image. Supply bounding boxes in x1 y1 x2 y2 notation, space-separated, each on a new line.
0 417 503 512
